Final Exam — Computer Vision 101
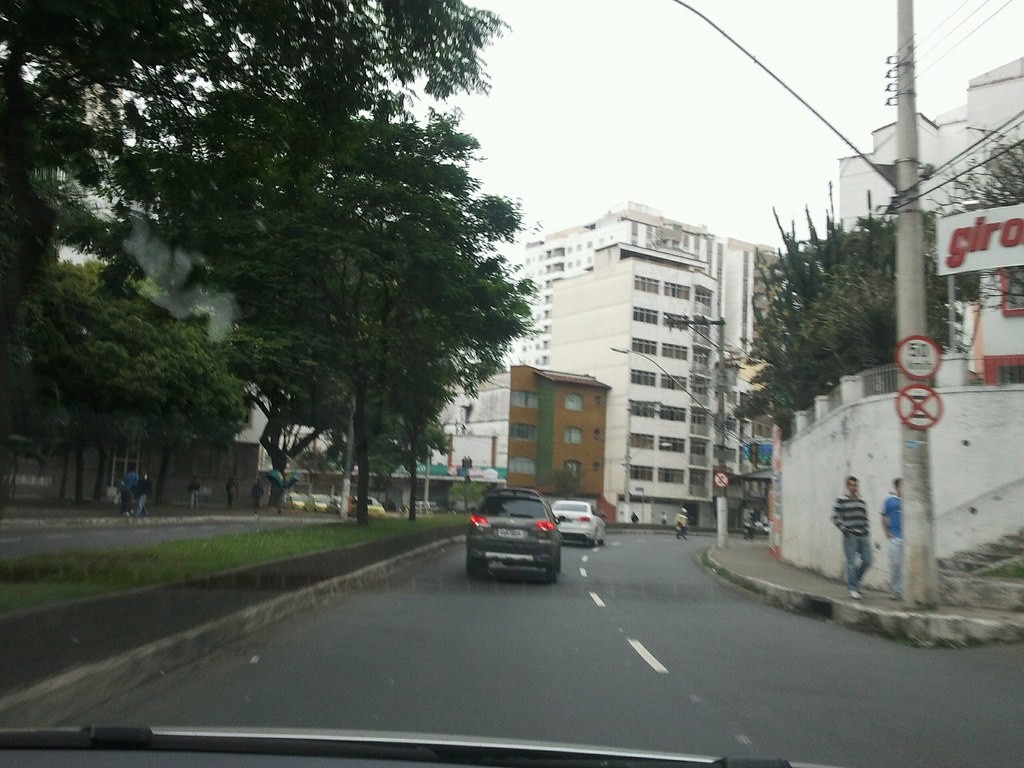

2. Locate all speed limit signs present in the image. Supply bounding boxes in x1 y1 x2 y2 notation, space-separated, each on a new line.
896 334 941 381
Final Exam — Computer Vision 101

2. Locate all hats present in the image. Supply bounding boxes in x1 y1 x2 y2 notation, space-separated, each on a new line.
682 508 687 513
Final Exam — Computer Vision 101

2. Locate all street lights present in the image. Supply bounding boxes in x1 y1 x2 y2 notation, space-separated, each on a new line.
608 344 728 548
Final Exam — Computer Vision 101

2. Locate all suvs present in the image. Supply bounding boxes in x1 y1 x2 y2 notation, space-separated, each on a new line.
465 486 567 586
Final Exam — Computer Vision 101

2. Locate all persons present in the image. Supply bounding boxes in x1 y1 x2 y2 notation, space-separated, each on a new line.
226 478 237 506
676 504 691 539
660 512 667 528
117 471 151 517
252 478 264 513
187 481 200 510
880 478 902 600
744 517 754 541
832 476 872 598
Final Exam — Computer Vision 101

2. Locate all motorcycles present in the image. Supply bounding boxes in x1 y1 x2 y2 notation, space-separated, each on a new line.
743 523 754 540
675 514 689 541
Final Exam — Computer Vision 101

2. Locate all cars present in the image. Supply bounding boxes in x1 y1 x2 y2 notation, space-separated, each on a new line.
438 501 479 515
412 501 438 514
286 491 387 517
549 500 608 548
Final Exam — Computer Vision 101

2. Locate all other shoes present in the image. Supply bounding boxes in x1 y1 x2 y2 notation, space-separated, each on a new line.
848 589 861 599
888 591 897 601
123 512 130 517
130 509 133 514
